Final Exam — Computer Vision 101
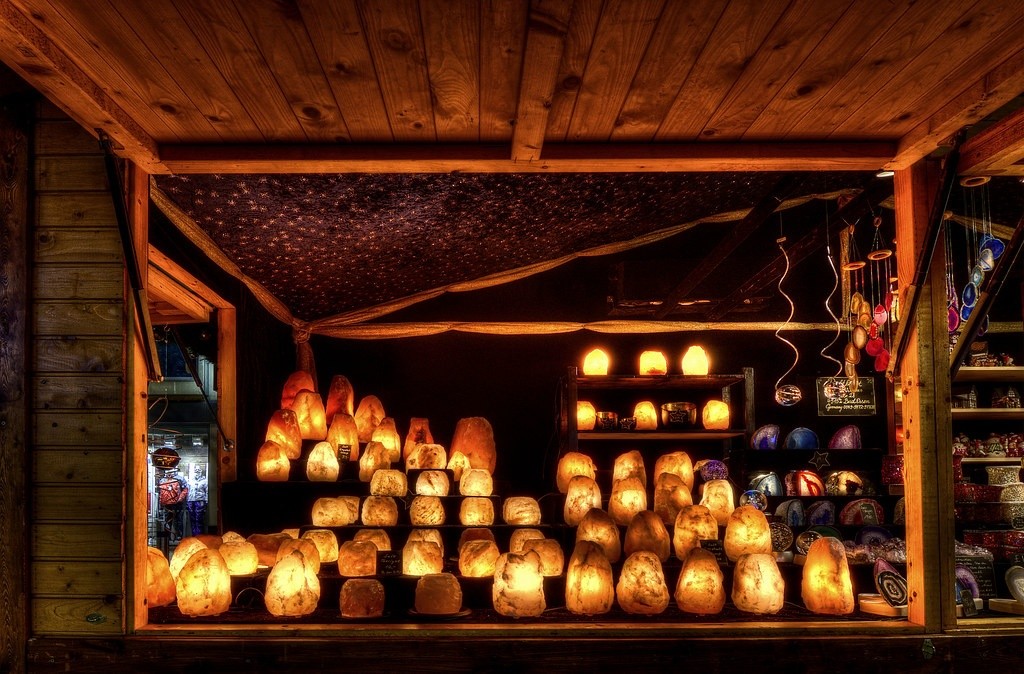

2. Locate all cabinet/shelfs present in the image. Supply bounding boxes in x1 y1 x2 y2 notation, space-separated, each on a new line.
237 439 566 614
950 322 1024 461
554 366 755 481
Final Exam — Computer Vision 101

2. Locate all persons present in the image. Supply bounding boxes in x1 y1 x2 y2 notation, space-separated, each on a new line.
188 468 208 536
159 466 188 541
